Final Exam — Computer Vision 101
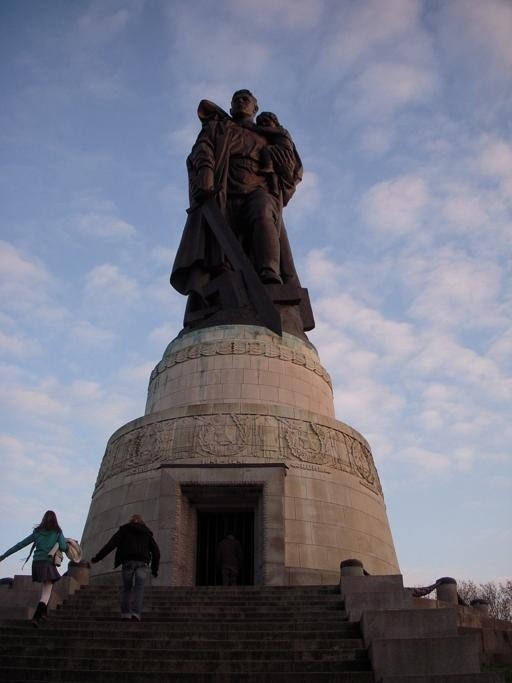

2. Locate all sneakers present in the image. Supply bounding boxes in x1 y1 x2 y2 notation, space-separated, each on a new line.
121 613 131 619
132 613 140 621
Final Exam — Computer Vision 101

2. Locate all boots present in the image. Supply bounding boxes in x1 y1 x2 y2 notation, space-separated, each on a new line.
30 602 47 628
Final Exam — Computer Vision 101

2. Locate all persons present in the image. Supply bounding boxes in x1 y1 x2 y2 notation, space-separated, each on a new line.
216 534 241 586
0 511 78 632
170 89 303 328
91 512 160 623
